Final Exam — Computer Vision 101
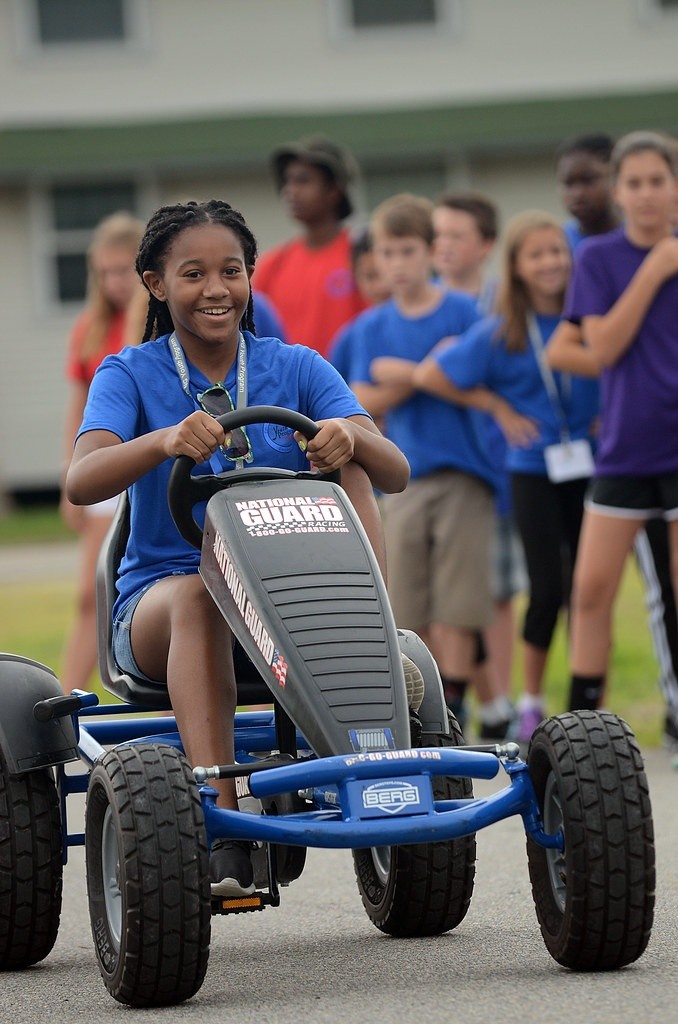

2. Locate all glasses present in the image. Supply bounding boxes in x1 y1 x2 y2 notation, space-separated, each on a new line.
557 170 605 189
277 170 321 190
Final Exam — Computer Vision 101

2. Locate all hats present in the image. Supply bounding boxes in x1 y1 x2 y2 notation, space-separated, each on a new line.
270 136 353 222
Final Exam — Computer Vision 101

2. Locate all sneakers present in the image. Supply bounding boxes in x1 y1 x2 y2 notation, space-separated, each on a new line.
400 652 425 741
207 827 256 897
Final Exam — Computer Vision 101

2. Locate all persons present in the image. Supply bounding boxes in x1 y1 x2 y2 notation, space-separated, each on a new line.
60 134 678 752
567 131 678 713
66 198 425 900
418 209 603 748
350 192 503 736
